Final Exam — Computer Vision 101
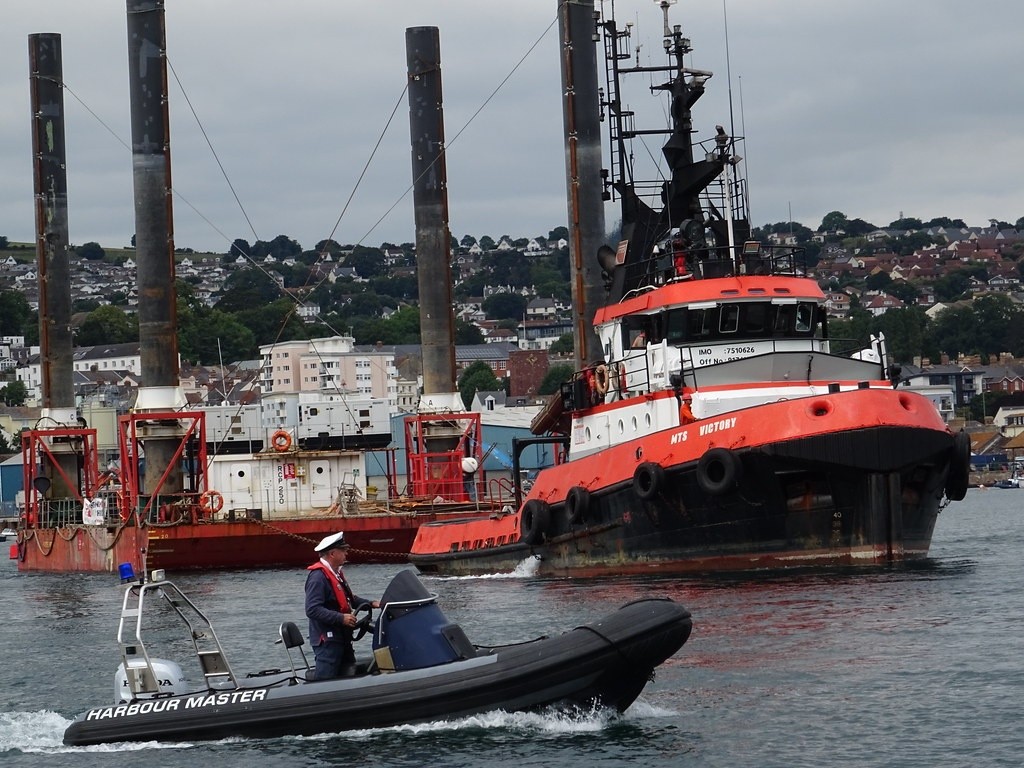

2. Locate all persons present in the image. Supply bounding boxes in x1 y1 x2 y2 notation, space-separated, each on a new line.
304 531 381 683
631 329 647 347
679 393 701 426
175 489 192 520
945 424 952 433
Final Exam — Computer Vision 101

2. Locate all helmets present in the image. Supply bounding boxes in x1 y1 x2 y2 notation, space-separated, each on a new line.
682 393 692 400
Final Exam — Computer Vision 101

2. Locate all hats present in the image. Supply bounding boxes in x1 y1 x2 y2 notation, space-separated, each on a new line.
314 531 350 553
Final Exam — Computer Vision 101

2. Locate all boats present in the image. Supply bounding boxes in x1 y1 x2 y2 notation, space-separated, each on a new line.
407 0 951 576
61 542 692 747
2 528 18 536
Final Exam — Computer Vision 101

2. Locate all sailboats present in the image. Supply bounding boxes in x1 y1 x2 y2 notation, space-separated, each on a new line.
995 447 1020 488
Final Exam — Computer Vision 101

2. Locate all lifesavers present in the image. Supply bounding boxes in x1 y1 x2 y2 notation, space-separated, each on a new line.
200 490 224 514
272 432 291 451
611 362 625 393
632 462 667 499
566 485 590 524
594 365 610 395
521 499 552 545
697 448 739 494
946 431 971 500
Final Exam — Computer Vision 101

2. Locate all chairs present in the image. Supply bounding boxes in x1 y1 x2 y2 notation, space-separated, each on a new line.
278 621 316 686
